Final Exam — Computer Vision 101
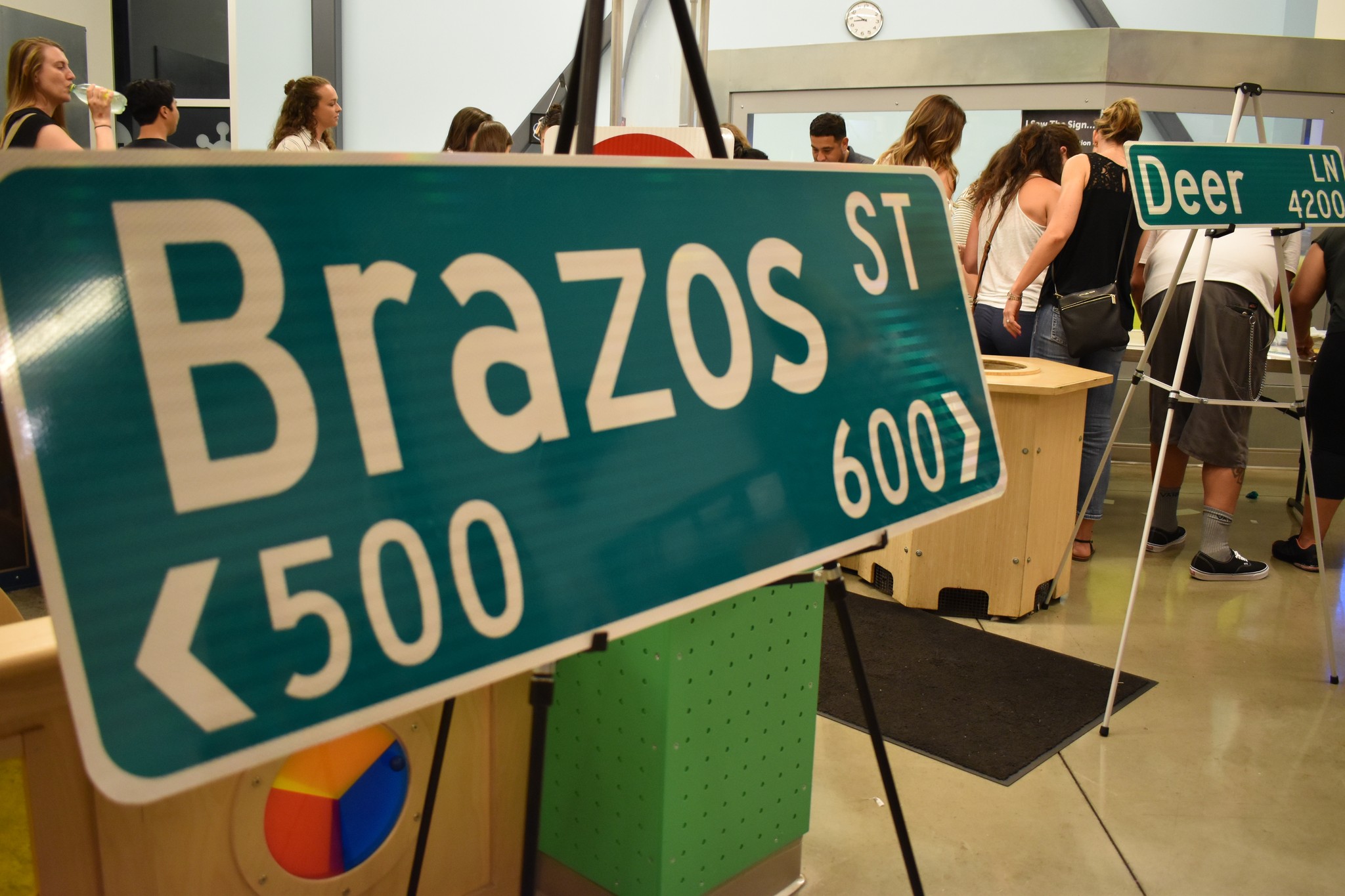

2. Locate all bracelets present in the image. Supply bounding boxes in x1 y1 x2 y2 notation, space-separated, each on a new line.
94 125 112 129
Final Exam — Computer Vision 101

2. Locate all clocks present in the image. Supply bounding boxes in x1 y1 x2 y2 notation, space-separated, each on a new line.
845 0 884 40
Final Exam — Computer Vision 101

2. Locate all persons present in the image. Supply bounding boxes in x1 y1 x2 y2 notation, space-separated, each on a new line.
720 123 768 160
873 94 1081 357
0 37 116 150
809 113 875 164
266 76 342 151
1271 227 1345 570
123 79 183 148
1000 98 1142 563
1131 228 1303 582
539 104 563 153
470 121 514 153
441 107 493 152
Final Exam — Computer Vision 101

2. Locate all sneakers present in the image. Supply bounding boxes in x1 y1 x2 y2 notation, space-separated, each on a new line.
1270 535 1320 570
1189 548 1268 579
1145 525 1187 552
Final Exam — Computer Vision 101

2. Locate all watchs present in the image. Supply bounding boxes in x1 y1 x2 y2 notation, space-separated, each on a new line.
1006 291 1023 301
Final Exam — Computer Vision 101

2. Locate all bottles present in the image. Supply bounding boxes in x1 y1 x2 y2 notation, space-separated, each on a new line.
69 83 128 114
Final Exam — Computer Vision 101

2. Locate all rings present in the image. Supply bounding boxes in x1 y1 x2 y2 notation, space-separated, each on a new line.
1006 320 1010 323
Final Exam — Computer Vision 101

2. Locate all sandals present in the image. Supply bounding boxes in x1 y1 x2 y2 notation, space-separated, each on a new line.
1071 539 1093 561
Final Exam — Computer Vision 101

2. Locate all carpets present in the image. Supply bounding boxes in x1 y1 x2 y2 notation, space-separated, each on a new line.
816 585 1158 786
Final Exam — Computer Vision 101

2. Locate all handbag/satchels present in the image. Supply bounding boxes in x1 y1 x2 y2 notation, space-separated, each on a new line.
1058 283 1130 358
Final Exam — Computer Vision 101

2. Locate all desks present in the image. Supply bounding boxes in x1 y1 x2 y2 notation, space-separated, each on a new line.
1123 330 1325 515
